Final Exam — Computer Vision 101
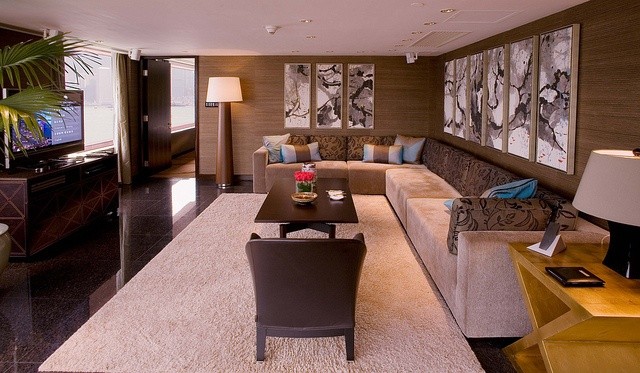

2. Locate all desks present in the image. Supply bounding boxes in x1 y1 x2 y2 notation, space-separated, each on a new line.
0 150 119 262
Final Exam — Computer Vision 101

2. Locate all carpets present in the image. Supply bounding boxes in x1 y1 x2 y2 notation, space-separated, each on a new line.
37 194 487 373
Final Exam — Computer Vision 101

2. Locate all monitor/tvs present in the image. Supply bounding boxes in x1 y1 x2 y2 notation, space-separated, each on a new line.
2 88 85 173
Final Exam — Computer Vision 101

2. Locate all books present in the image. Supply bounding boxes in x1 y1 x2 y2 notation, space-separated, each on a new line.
544 266 606 288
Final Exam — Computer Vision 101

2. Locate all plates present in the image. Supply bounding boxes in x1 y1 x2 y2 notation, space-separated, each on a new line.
328 190 344 200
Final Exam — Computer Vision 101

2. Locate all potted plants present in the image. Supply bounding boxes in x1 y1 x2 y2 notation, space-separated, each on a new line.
0 30 103 274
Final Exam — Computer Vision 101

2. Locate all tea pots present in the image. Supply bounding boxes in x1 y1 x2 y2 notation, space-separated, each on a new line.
300 162 318 187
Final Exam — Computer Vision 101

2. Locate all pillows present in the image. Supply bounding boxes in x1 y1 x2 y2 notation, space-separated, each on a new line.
480 178 538 199
281 142 322 164
444 200 453 211
362 143 403 165
394 133 426 165
262 132 291 164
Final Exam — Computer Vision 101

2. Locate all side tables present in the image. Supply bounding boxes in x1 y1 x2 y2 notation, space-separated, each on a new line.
501 242 640 373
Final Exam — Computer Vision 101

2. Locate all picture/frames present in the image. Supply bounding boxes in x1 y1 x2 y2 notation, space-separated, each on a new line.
455 55 467 140
484 43 510 154
467 50 484 146
346 64 375 130
283 62 311 130
316 63 344 130
443 59 455 136
507 34 540 163
534 23 581 175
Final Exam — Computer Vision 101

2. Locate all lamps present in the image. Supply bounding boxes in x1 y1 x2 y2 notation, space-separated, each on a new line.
205 76 244 187
43 28 57 43
405 52 418 64
128 49 140 61
571 147 640 280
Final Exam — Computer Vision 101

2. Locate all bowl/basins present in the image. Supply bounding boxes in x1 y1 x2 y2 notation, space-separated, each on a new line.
291 192 318 203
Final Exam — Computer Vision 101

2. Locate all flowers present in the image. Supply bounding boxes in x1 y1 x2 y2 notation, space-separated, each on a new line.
294 171 315 181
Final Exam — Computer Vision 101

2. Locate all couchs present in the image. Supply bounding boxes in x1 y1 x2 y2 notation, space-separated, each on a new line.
252 133 427 195
385 143 610 339
244 232 367 361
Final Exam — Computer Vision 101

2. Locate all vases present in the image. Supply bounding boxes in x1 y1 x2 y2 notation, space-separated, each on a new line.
295 181 313 193
